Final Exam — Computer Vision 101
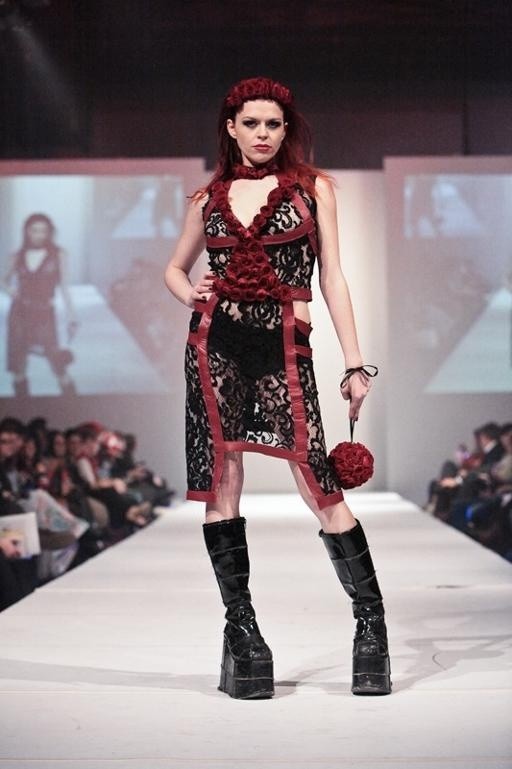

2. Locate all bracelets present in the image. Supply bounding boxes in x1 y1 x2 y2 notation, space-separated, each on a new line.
343 365 364 373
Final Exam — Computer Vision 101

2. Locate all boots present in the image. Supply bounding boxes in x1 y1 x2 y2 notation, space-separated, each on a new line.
318 518 392 698
201 514 277 700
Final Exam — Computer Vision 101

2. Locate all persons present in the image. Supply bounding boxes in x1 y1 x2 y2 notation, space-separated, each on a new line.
2 414 176 601
0 212 83 405
157 76 392 701
418 416 510 561
